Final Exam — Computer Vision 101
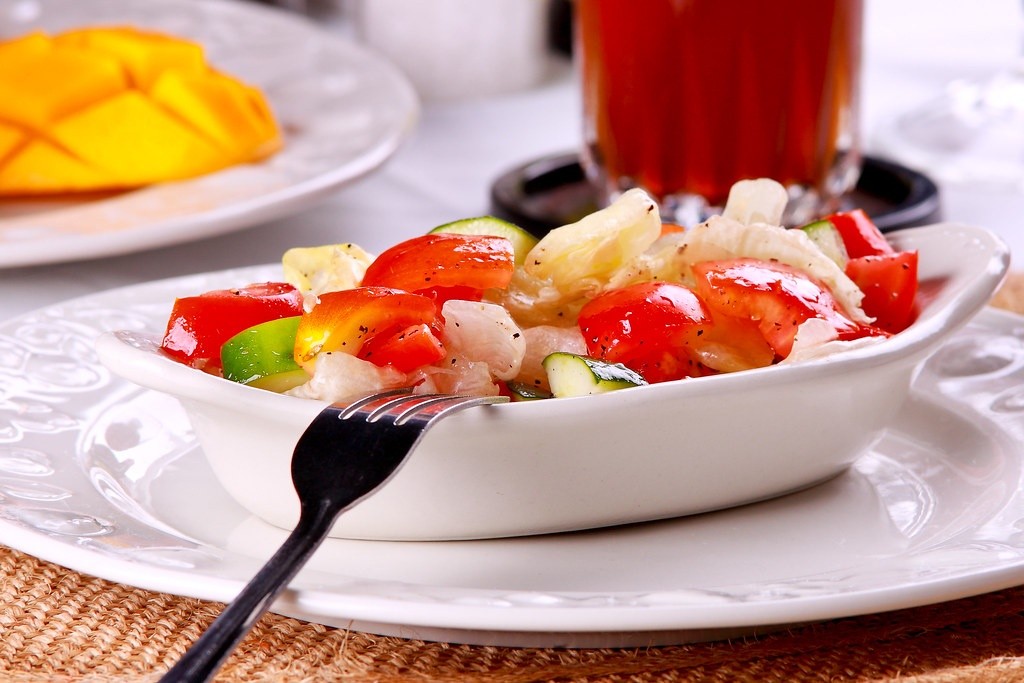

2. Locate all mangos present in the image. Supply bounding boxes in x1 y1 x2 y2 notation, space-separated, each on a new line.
0 25 283 197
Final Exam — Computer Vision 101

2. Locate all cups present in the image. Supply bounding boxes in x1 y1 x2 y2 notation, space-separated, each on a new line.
572 1 864 223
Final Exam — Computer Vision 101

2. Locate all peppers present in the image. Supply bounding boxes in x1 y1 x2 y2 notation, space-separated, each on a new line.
158 208 920 376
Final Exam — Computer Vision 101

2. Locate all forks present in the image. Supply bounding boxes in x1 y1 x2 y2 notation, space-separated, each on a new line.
157 389 511 683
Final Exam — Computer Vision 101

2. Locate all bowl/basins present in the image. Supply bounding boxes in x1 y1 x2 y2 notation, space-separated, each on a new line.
65 221 1011 540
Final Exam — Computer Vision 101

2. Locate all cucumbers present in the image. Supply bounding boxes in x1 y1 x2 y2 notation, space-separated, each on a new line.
507 351 648 401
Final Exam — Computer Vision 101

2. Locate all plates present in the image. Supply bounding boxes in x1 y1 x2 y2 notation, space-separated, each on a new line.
1 1 418 266
494 148 942 233
0 256 1024 630
